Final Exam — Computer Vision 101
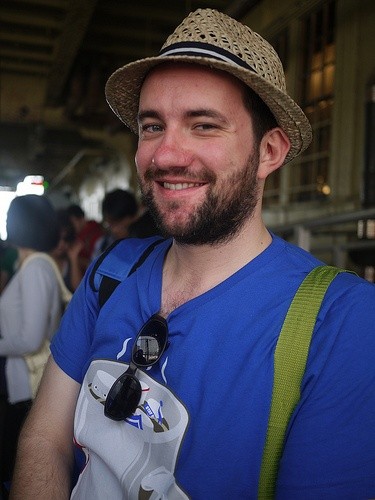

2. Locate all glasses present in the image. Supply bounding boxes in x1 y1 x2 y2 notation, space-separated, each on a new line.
104 316 167 422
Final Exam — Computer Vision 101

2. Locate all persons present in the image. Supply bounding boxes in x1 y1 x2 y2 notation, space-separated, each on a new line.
12 9 375 500
0 189 138 446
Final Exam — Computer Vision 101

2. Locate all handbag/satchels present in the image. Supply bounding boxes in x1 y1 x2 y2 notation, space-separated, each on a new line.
26 342 51 401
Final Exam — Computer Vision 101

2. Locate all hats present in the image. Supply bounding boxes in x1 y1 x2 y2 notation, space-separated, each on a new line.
104 8 311 167
